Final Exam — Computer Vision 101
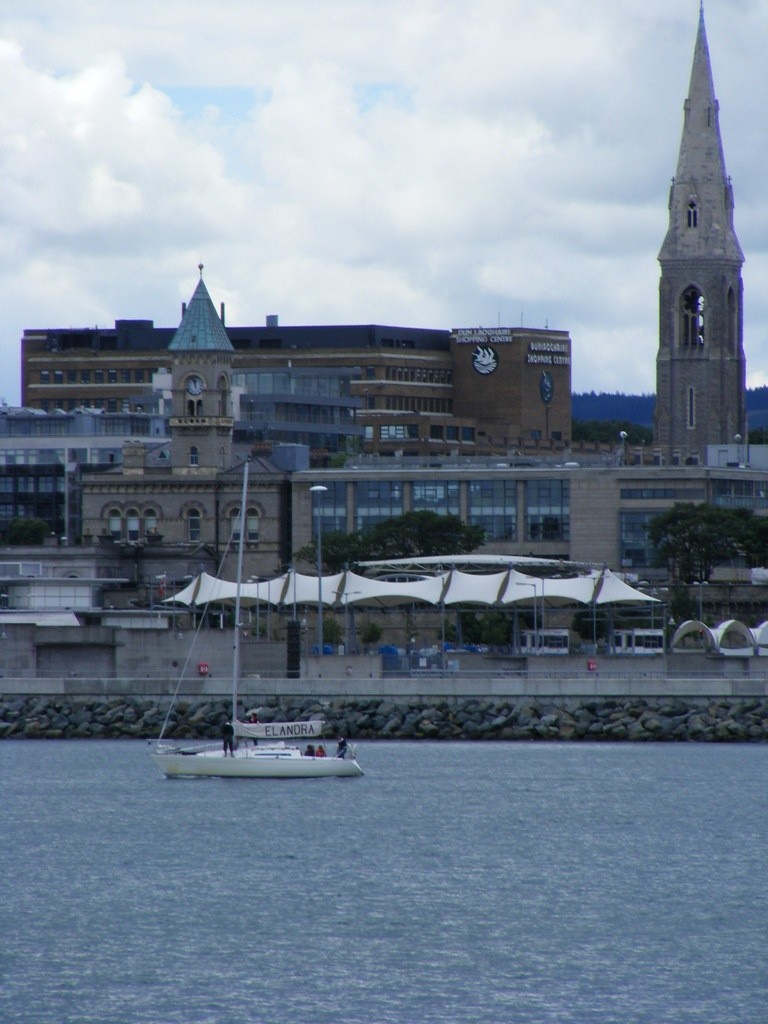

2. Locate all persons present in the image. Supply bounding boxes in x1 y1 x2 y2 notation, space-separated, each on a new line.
305 745 315 756
222 721 235 758
336 734 348 759
315 744 327 757
245 713 260 746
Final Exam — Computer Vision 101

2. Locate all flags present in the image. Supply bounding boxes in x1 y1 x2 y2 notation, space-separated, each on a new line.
159 575 167 596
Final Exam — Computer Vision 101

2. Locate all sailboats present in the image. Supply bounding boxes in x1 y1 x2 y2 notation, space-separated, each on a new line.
149 462 365 778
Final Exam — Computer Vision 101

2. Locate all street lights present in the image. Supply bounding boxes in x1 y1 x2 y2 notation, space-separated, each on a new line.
528 574 545 656
184 575 197 630
155 574 176 631
252 575 271 642
246 579 260 641
693 580 708 622
288 569 297 620
309 485 329 656
517 583 537 655
